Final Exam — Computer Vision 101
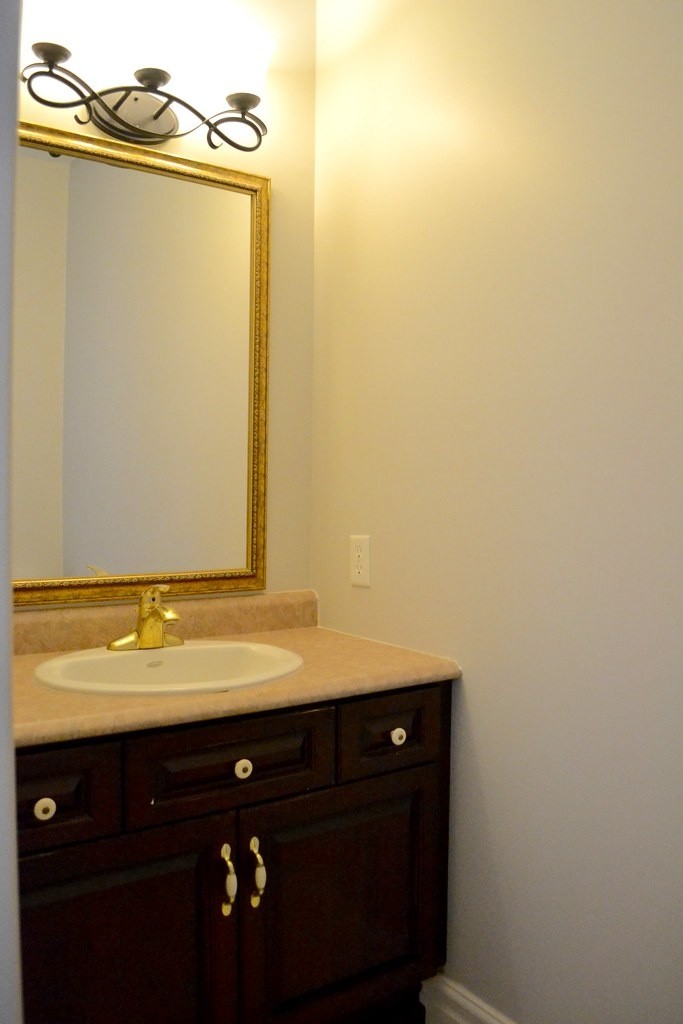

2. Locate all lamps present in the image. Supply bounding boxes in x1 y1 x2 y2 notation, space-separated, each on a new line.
19 41 269 153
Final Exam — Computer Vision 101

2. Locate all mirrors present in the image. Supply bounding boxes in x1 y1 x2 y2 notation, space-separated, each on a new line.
9 119 273 606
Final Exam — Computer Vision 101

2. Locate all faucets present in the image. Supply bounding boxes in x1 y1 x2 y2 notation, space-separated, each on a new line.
88 566 107 577
108 584 185 650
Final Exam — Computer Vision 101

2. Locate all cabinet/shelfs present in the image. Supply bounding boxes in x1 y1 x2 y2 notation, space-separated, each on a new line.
10 588 463 1024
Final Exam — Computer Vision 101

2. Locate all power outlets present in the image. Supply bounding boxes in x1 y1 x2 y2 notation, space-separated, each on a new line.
349 535 373 588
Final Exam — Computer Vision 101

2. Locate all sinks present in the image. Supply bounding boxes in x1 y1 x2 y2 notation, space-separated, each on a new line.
36 641 303 694
